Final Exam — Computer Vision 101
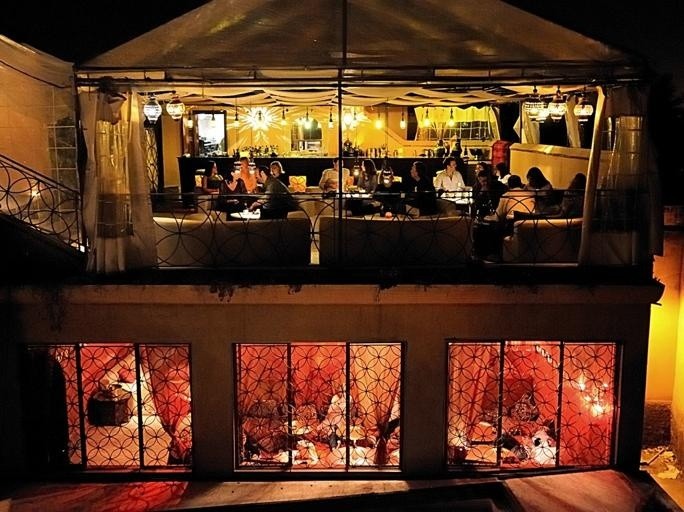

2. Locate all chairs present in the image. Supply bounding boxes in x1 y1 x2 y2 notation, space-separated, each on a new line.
151 174 579 262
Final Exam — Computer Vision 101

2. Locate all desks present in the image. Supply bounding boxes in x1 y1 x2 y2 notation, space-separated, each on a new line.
90 387 133 427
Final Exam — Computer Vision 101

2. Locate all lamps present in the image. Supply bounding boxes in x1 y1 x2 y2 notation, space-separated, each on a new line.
135 85 599 131
